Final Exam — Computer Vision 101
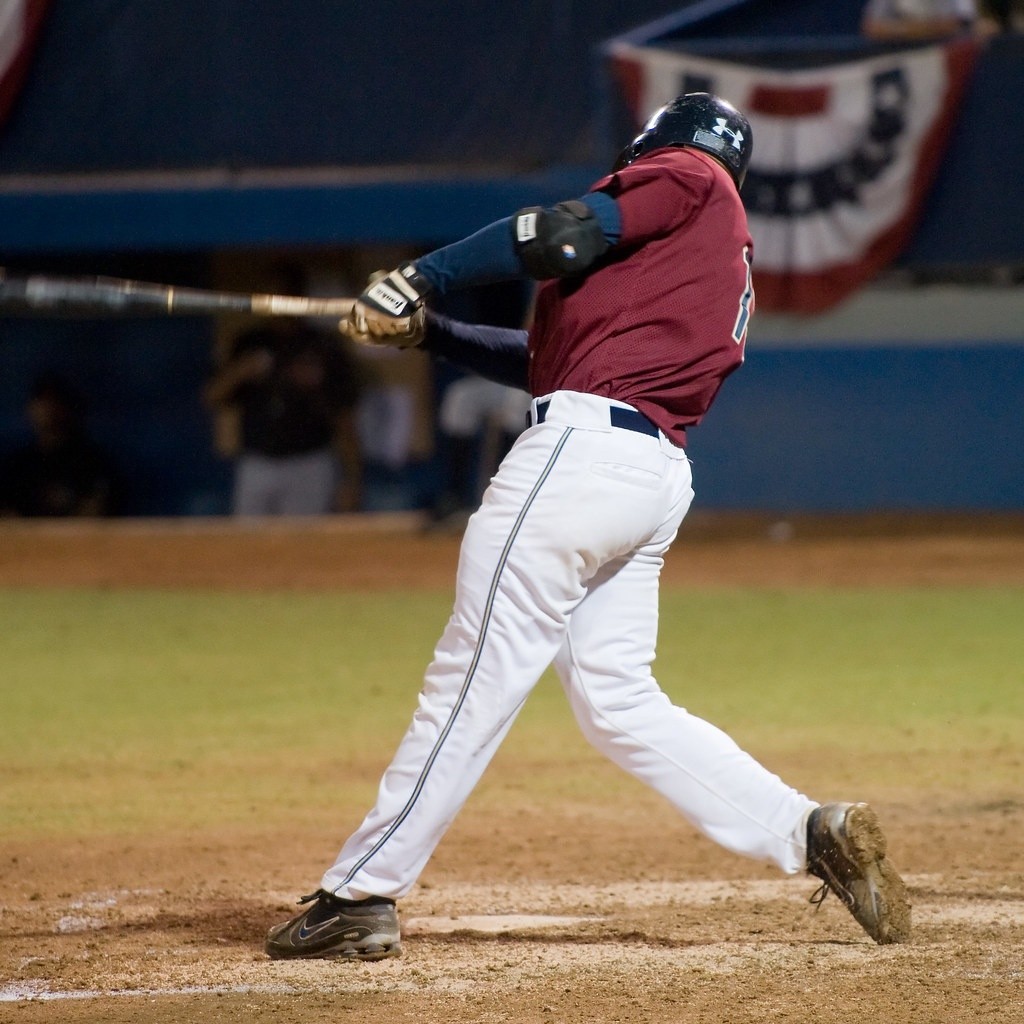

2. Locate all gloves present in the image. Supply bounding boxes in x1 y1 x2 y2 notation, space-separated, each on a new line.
337 259 427 352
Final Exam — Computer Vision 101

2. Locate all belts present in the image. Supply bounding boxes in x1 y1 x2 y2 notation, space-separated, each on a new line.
525 401 659 439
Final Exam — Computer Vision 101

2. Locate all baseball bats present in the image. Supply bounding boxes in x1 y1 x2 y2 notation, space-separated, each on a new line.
1 271 426 322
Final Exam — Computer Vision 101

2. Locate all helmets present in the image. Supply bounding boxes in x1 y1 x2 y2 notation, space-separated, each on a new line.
613 92 753 194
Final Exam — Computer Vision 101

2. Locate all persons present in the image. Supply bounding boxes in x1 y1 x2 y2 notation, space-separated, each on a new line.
2 264 415 519
264 93 913 961
860 0 1018 286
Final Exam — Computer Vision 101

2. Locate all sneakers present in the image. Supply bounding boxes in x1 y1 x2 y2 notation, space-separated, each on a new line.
805 801 912 944
263 889 403 961
